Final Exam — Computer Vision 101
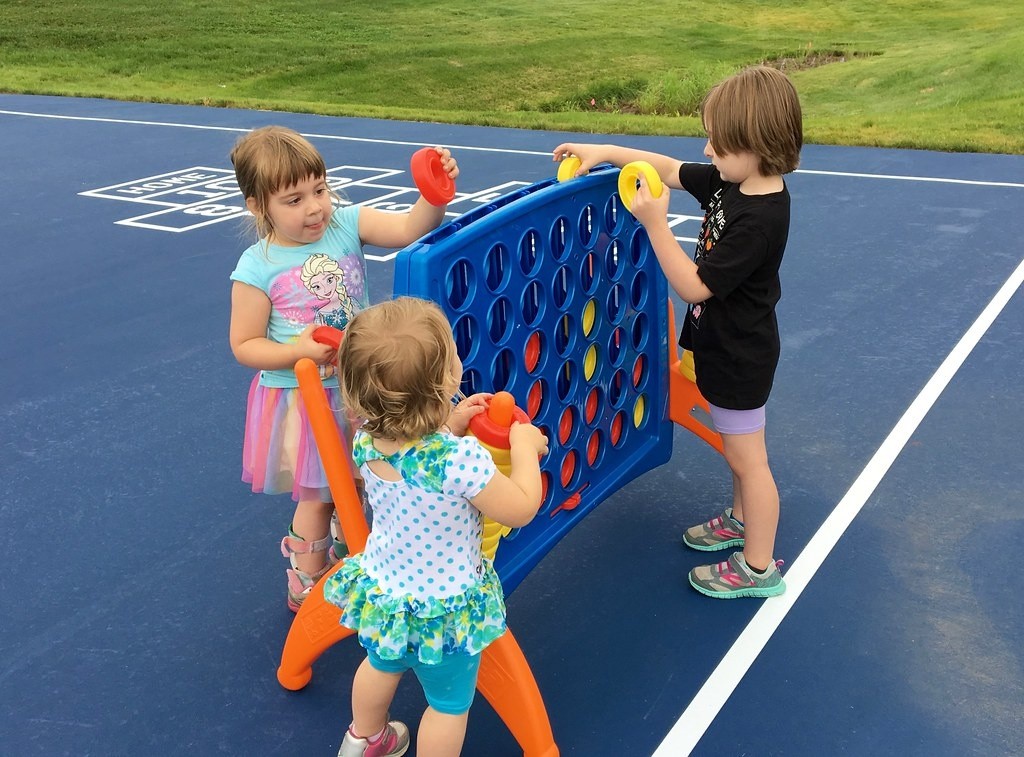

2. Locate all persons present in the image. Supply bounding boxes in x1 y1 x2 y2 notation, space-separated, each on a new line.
334 295 548 757
553 64 804 599
223 124 461 611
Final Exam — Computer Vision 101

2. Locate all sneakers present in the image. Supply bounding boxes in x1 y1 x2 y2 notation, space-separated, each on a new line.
683 508 745 552
328 545 350 566
338 713 410 757
286 562 335 614
688 552 786 599
